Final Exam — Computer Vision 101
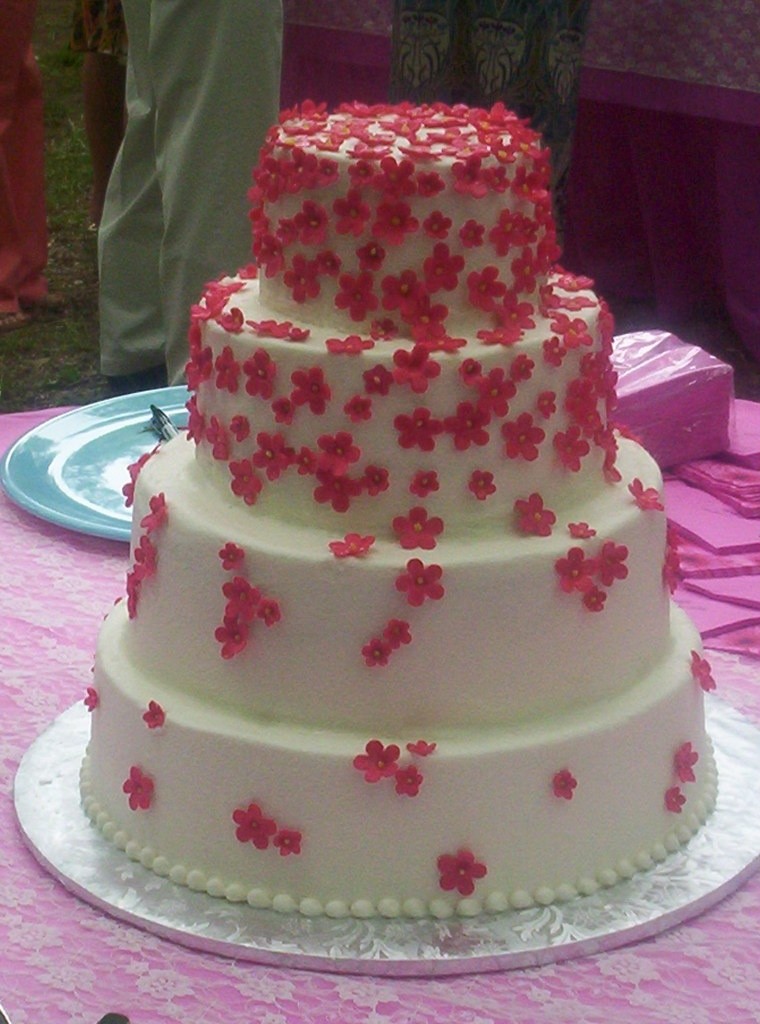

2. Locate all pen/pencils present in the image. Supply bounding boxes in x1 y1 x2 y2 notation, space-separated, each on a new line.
146 401 178 440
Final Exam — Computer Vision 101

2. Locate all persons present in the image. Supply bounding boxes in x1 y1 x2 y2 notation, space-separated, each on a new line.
99 0 285 376
70 0 129 225
0 0 65 332
386 0 591 248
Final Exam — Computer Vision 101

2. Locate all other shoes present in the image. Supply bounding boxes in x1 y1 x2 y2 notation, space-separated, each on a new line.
0 291 71 332
109 364 168 391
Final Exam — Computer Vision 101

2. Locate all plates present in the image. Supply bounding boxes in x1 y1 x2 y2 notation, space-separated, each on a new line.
0 385 192 542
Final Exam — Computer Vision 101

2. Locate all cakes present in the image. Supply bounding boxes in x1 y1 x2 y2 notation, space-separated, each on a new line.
77 101 720 918
